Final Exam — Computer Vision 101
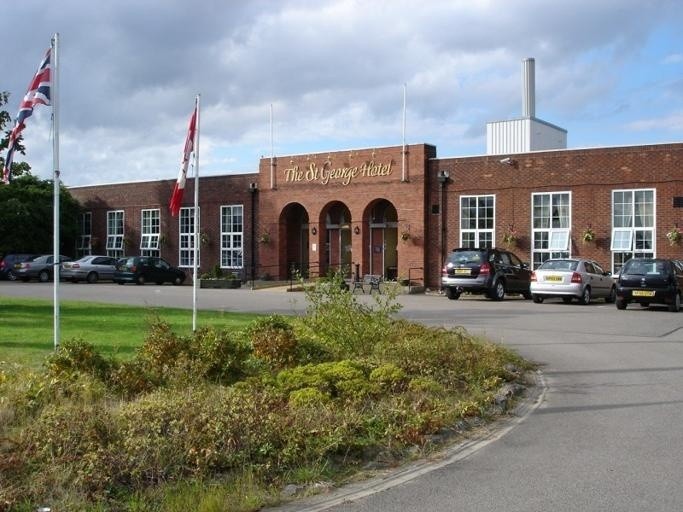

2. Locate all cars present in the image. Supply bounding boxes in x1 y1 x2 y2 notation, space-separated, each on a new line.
60 255 118 284
112 255 186 285
0 254 73 282
615 259 683 310
529 258 616 305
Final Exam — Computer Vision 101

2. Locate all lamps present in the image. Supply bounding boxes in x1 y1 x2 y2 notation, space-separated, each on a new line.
311 227 317 235
354 226 360 235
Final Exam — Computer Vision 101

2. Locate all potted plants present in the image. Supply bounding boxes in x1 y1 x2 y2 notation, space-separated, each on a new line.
200 263 242 289
581 227 597 244
385 232 398 282
667 228 683 247
501 227 519 248
401 232 410 241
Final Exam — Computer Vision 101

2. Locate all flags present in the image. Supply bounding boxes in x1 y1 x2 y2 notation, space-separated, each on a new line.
166 99 197 218
0 40 52 188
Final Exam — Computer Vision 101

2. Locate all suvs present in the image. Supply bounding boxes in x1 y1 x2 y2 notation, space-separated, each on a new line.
443 248 533 301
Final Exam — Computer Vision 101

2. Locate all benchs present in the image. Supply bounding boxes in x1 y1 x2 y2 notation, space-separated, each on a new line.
352 274 382 295
